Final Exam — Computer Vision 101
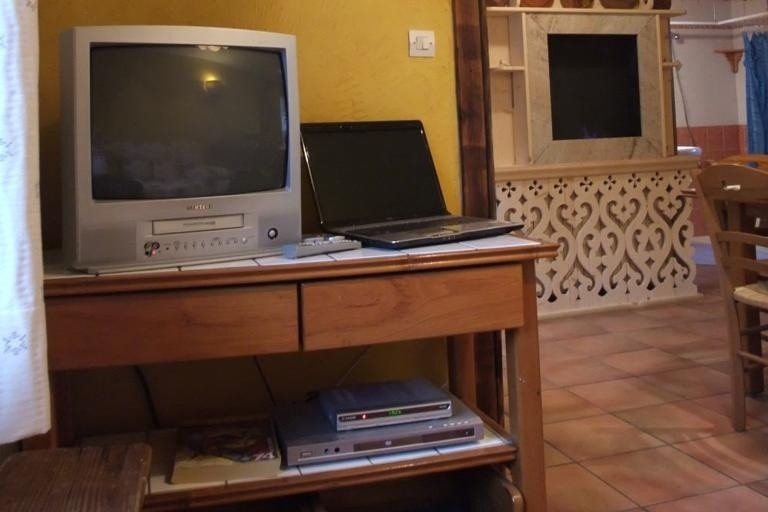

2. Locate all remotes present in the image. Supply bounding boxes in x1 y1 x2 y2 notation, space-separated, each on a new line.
285 237 362 257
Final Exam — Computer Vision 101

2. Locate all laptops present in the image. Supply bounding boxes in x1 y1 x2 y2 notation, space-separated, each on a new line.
301 119 524 249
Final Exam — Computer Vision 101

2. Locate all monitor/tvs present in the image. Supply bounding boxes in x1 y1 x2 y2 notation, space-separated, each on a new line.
61 25 300 278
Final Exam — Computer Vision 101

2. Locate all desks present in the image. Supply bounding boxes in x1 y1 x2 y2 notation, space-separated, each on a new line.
21 233 560 512
680 184 766 396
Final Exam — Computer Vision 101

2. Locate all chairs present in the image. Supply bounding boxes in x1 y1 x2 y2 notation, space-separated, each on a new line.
693 164 768 432
719 155 768 173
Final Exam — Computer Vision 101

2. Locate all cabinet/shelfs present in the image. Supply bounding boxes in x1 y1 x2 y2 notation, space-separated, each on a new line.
485 5 687 167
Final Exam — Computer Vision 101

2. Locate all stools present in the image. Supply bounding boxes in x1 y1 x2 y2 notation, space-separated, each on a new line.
0 442 152 512
